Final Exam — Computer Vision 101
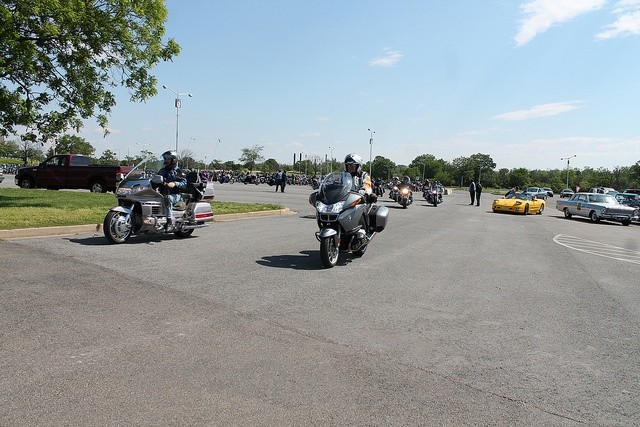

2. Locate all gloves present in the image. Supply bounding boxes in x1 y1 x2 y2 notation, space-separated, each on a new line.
359 189 365 196
168 182 175 188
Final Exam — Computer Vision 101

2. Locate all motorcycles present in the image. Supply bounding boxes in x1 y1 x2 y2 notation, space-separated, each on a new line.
215 170 308 185
308 170 389 266
391 185 400 201
421 186 432 197
396 185 414 209
375 185 386 196
430 187 444 206
103 155 215 243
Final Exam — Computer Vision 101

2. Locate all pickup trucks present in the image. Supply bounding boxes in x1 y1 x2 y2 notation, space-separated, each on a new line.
14 154 135 191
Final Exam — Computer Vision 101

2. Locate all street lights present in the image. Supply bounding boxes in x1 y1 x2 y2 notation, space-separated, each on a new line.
561 155 577 189
136 142 153 178
163 84 193 151
328 145 335 173
368 128 376 177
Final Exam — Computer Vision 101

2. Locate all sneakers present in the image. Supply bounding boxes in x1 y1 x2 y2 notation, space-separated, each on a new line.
163 218 172 227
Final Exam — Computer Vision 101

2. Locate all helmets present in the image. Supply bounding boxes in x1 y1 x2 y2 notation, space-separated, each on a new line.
161 150 178 165
345 153 362 165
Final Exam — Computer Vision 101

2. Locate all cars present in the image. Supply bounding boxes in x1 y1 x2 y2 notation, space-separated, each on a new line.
543 187 553 196
522 187 547 199
560 188 574 198
556 192 640 225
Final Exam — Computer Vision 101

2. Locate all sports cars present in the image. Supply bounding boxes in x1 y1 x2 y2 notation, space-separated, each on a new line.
492 192 546 214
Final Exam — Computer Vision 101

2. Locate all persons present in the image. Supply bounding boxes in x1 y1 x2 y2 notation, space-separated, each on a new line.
283 170 287 192
287 171 326 190
469 179 476 205
396 176 414 202
422 180 432 196
374 177 385 193
200 168 275 185
476 179 482 206
502 185 518 199
387 176 401 198
428 180 442 198
335 154 370 243
275 170 283 192
147 150 187 229
